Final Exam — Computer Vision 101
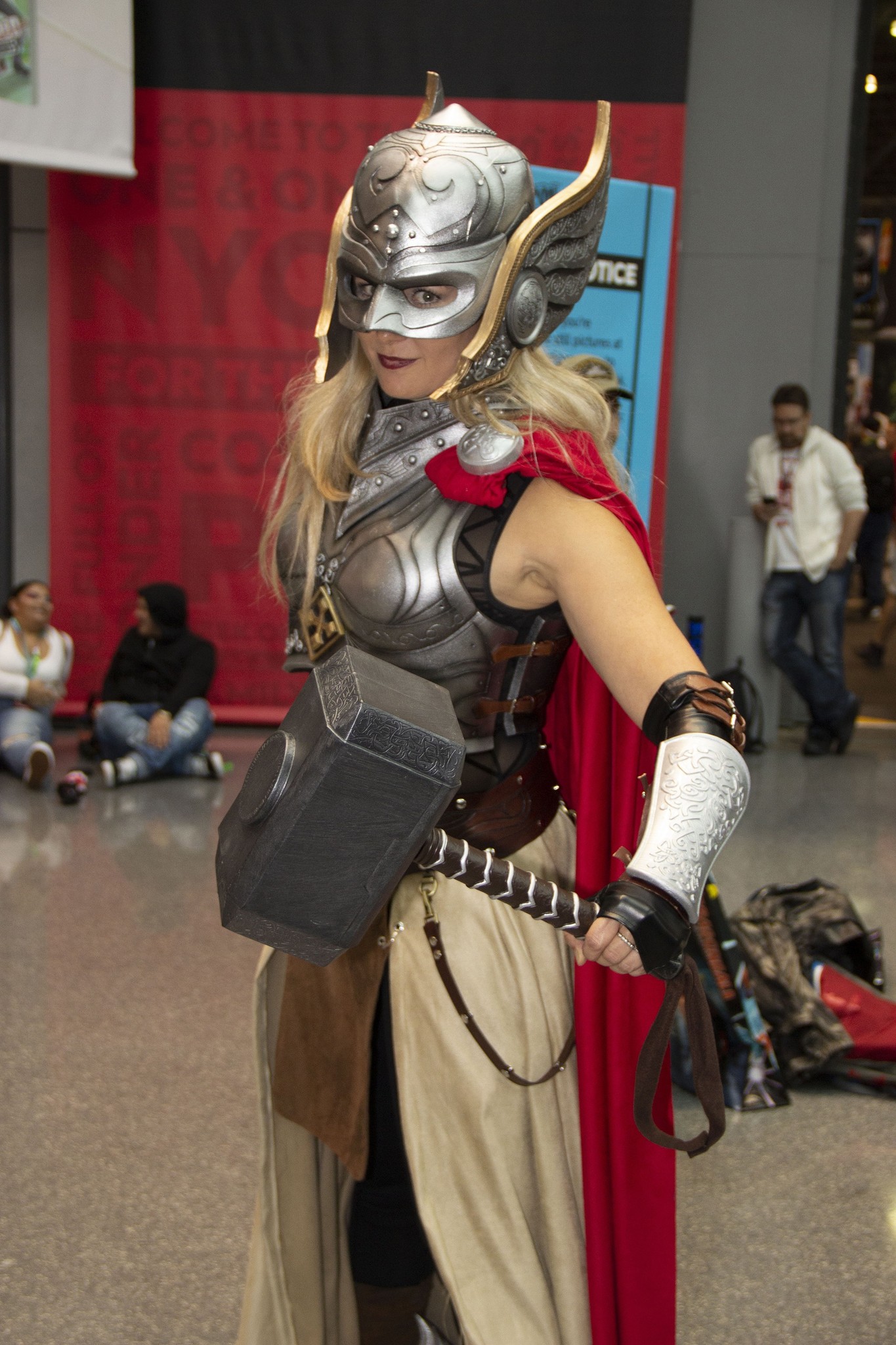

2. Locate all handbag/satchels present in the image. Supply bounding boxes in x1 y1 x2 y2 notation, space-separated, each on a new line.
664 877 896 1111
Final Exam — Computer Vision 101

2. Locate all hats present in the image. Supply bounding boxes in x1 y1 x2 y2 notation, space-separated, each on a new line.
560 356 634 402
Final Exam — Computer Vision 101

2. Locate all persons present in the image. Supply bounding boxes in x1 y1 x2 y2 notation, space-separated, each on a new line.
92 582 225 785
557 355 635 451
746 383 867 755
233 73 754 1344
0 578 74 785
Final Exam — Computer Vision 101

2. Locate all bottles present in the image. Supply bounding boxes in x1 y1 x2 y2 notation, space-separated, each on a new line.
24 647 42 679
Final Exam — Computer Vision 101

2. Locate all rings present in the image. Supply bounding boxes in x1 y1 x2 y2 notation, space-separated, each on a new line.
618 933 639 954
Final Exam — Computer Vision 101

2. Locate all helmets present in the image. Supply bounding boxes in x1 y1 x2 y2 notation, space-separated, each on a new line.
316 66 611 406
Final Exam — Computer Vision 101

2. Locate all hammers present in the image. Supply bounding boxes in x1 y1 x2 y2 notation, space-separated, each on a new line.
215 647 687 976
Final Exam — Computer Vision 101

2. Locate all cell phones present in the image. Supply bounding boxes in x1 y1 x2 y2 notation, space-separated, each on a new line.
764 498 775 503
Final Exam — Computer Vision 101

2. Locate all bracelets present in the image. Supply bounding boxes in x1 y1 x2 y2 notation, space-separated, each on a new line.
158 709 172 720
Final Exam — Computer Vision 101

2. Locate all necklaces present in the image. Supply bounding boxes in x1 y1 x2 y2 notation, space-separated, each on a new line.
11 618 45 680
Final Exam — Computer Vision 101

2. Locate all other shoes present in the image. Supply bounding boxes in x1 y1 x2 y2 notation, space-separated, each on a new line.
102 759 121 787
802 718 836 756
23 738 56 791
196 751 225 780
834 693 863 757
862 606 882 619
